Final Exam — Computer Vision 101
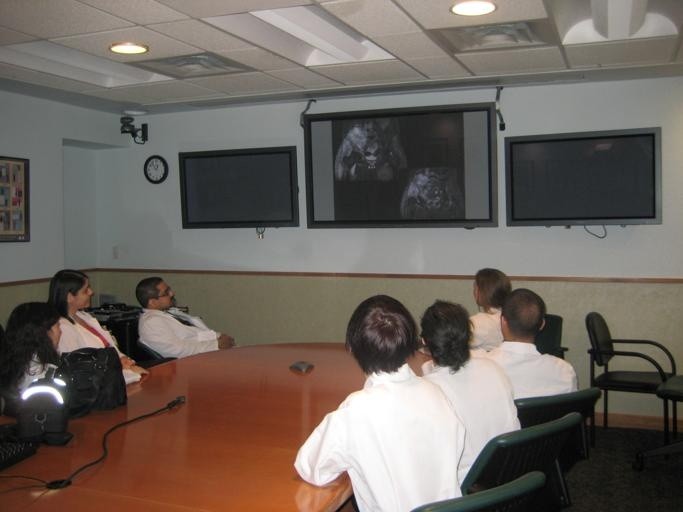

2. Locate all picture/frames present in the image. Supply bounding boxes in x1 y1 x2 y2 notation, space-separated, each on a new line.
0 159 36 244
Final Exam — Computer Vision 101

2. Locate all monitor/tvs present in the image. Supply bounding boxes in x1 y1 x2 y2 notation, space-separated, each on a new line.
503 126 663 228
177 144 300 229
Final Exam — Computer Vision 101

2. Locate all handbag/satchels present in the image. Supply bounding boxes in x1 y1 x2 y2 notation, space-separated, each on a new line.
52 346 129 420
15 376 74 447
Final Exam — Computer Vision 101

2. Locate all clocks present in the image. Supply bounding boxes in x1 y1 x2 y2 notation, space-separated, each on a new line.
145 154 168 185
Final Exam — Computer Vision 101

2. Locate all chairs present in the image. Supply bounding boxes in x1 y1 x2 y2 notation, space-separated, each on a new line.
659 364 683 442
133 335 180 365
514 383 611 507
416 469 558 512
587 309 675 437
460 411 594 494
541 316 572 363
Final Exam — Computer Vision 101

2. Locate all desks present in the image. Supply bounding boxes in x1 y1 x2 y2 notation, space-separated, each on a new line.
2 340 418 510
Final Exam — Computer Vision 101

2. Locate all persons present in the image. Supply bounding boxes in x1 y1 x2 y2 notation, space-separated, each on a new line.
418 299 524 472
483 287 578 399
293 293 467 512
468 268 516 354
0 301 63 426
45 268 150 386
135 276 239 359
